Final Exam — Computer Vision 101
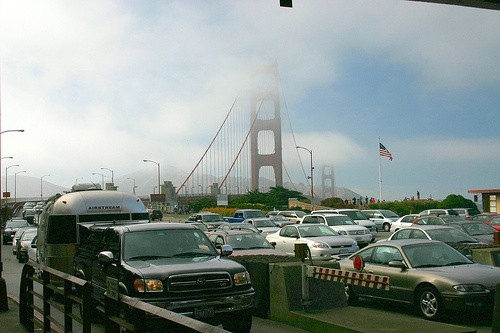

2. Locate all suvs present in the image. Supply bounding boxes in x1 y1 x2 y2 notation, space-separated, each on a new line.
73 220 256 333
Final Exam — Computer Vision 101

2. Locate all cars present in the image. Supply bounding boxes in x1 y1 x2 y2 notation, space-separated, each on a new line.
328 238 500 321
185 207 500 263
2 187 164 302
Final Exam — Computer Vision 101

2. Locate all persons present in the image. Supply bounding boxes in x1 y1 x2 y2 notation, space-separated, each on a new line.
345 196 375 205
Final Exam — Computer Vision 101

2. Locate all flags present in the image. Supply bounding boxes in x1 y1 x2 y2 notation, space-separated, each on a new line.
380 143 392 161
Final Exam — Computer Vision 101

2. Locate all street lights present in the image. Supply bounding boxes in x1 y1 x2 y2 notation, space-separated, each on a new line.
143 160 160 194
5 164 20 207
296 146 314 199
100 167 114 191
126 177 136 194
92 172 105 190
13 170 27 204
40 175 50 201
